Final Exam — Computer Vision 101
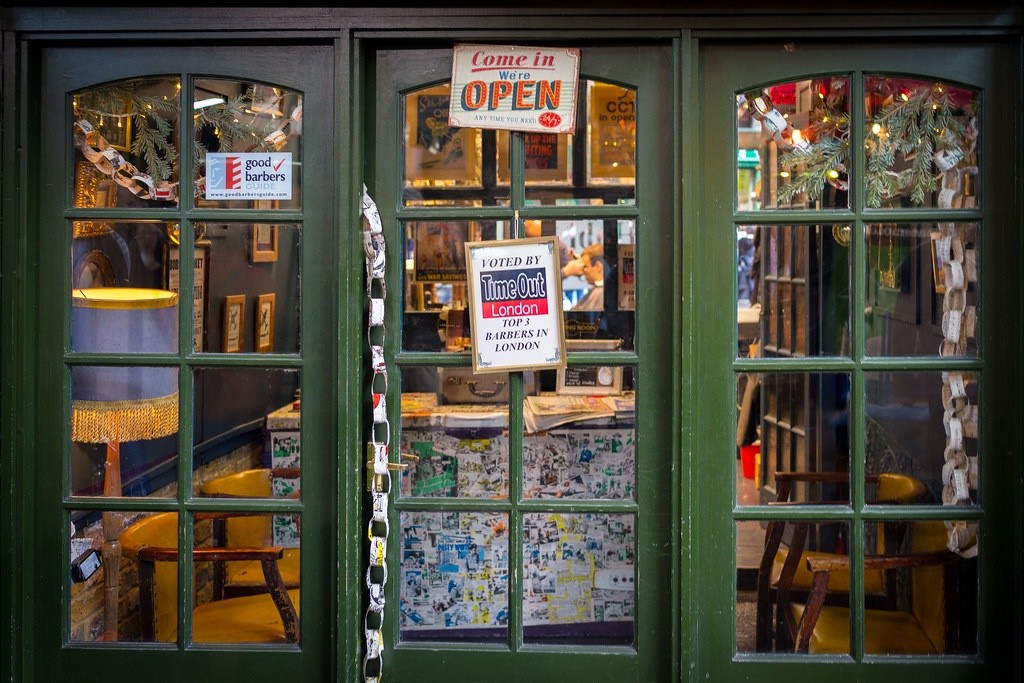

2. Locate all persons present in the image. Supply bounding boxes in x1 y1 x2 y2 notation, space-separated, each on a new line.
513 220 584 283
569 242 605 312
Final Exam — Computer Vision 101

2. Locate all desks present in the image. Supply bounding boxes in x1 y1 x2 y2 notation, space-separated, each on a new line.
264 392 636 644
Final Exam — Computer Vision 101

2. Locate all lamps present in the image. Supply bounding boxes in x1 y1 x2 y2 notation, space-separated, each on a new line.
70 287 179 641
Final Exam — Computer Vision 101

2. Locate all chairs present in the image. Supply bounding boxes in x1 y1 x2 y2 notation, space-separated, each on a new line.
199 468 301 602
757 472 925 653
775 519 977 654
119 511 300 644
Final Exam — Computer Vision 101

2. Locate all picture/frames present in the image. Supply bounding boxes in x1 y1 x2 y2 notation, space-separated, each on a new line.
248 200 279 263
463 236 567 376
223 294 245 353
73 161 118 240
255 292 276 353
414 221 475 284
555 338 623 396
401 78 635 200
74 96 132 153
